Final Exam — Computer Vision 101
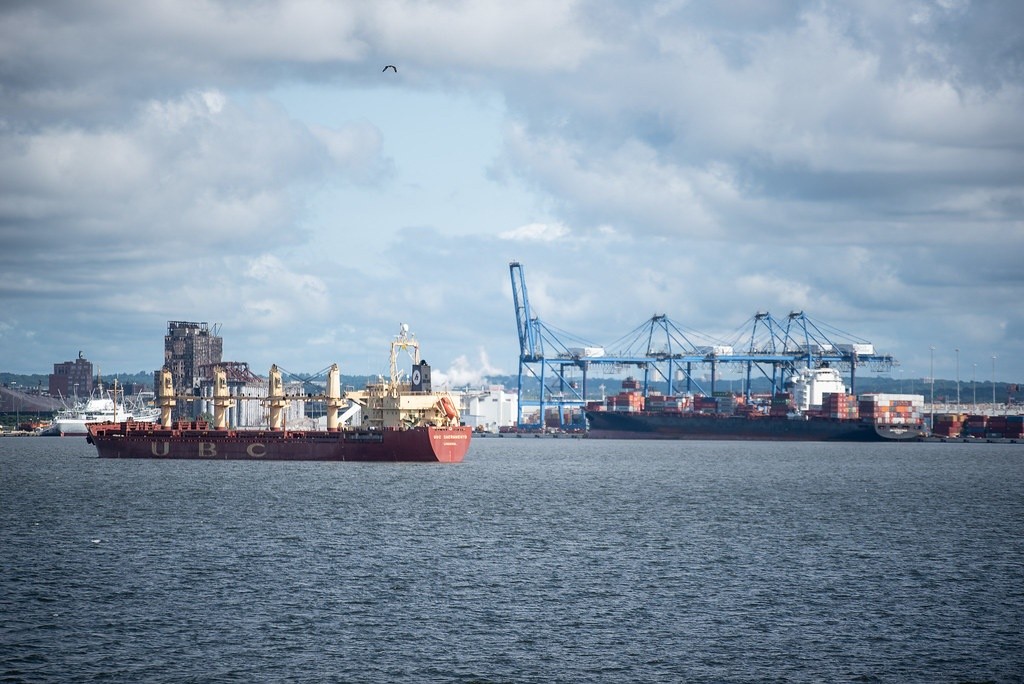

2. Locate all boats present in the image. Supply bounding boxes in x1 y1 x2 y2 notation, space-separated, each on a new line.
55 381 157 437
84 323 472 464
580 364 928 442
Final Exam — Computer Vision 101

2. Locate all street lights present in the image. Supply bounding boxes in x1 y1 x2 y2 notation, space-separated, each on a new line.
929 346 936 429
993 356 999 415
972 362 979 408
954 349 960 410
